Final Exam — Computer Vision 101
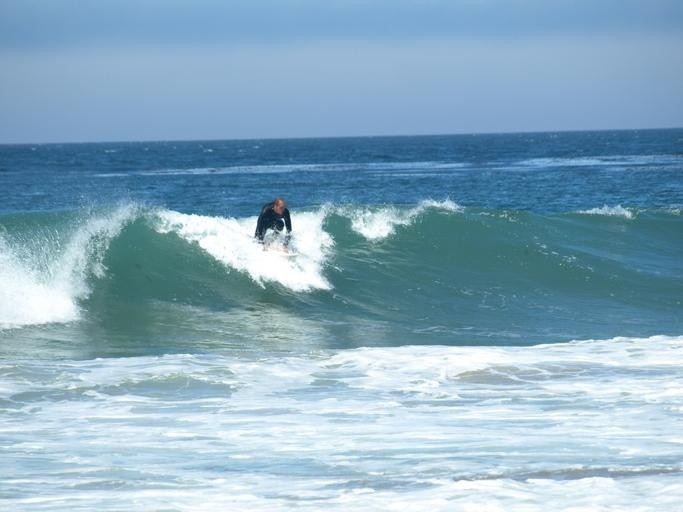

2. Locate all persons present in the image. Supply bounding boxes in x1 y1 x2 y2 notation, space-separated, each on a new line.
255 198 292 248
